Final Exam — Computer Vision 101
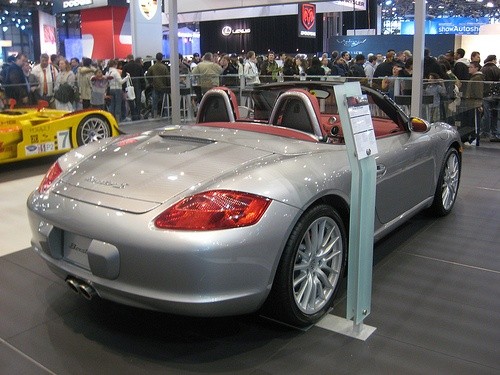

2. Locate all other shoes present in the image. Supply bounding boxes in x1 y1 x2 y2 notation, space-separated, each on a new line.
464 142 470 145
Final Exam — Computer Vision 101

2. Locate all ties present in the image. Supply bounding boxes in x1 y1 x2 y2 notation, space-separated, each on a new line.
43 69 47 94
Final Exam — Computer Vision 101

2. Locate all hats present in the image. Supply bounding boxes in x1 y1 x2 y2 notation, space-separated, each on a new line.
446 49 454 55
386 53 395 58
82 58 92 66
195 53 199 57
356 55 366 61
368 53 374 60
157 53 165 60
231 53 238 57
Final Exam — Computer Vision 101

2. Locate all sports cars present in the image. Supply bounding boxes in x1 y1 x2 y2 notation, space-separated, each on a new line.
27 80 464 327
0 99 122 165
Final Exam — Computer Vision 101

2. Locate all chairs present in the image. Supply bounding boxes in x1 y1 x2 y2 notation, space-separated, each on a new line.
269 88 333 143
195 87 241 124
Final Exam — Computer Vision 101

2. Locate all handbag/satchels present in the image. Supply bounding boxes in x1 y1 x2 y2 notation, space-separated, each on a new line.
125 77 136 100
54 82 74 103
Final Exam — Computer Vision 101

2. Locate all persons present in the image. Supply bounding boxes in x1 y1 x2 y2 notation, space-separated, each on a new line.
0 48 500 142
